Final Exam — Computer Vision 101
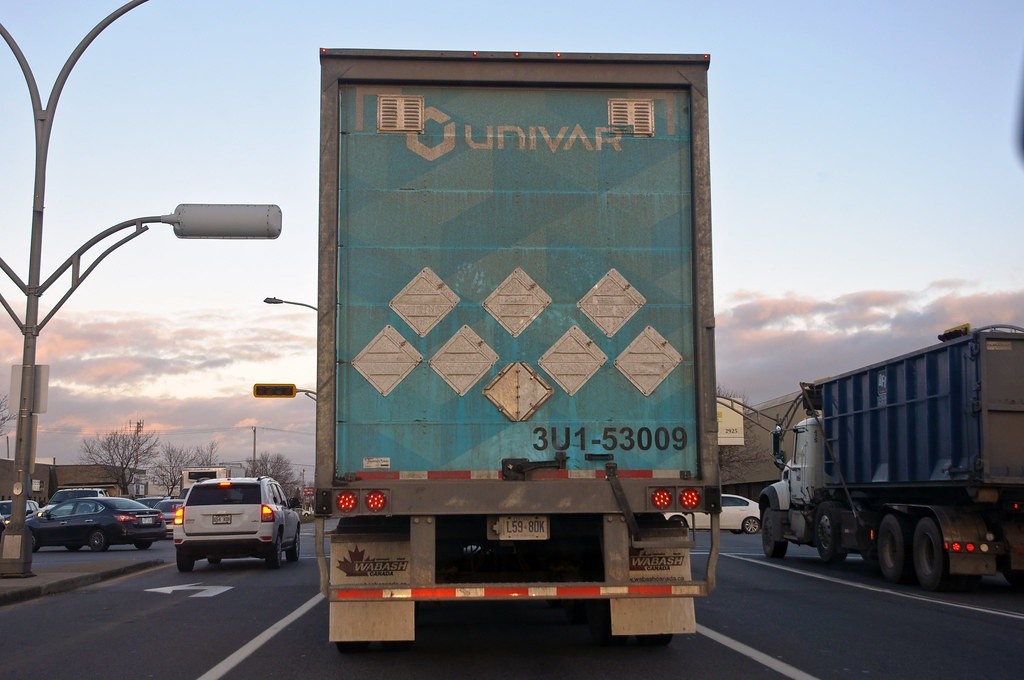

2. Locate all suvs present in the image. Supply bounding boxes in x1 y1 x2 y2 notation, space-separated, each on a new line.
38 487 110 517
173 476 301 572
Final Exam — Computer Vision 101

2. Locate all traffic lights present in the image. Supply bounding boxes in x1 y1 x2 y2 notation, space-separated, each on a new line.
253 383 296 398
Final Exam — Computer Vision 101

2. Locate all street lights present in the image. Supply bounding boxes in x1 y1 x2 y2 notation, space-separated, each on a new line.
263 297 318 312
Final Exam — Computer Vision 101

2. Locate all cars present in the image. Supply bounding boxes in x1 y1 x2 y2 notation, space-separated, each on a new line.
25 497 167 552
135 497 171 509
0 500 39 527
153 499 185 538
664 494 760 534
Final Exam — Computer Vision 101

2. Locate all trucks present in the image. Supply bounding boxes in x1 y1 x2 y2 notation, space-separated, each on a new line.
315 47 723 654
759 331 1024 592
